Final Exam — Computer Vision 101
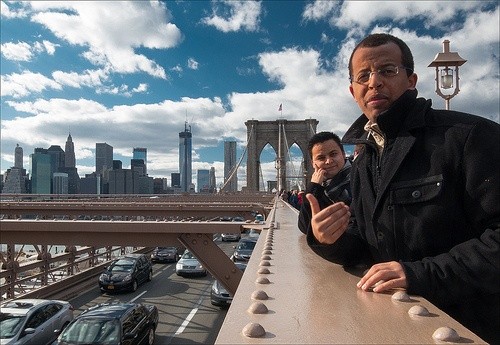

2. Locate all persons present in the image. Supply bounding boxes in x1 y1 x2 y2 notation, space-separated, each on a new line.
298 132 351 234
305 33 500 345
279 190 304 211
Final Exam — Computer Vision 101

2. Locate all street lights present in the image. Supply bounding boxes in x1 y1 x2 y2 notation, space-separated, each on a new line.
427 40 468 109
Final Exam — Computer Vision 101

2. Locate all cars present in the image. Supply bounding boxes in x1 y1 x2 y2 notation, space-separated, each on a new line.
219 214 265 239
211 262 248 308
99 253 153 291
150 245 187 263
175 245 208 277
233 236 258 262
0 296 72 345
47 302 159 344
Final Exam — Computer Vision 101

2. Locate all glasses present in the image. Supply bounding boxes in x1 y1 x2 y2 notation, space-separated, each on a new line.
352 66 414 85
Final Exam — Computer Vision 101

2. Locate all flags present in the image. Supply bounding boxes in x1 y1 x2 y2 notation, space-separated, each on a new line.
278 104 282 111
